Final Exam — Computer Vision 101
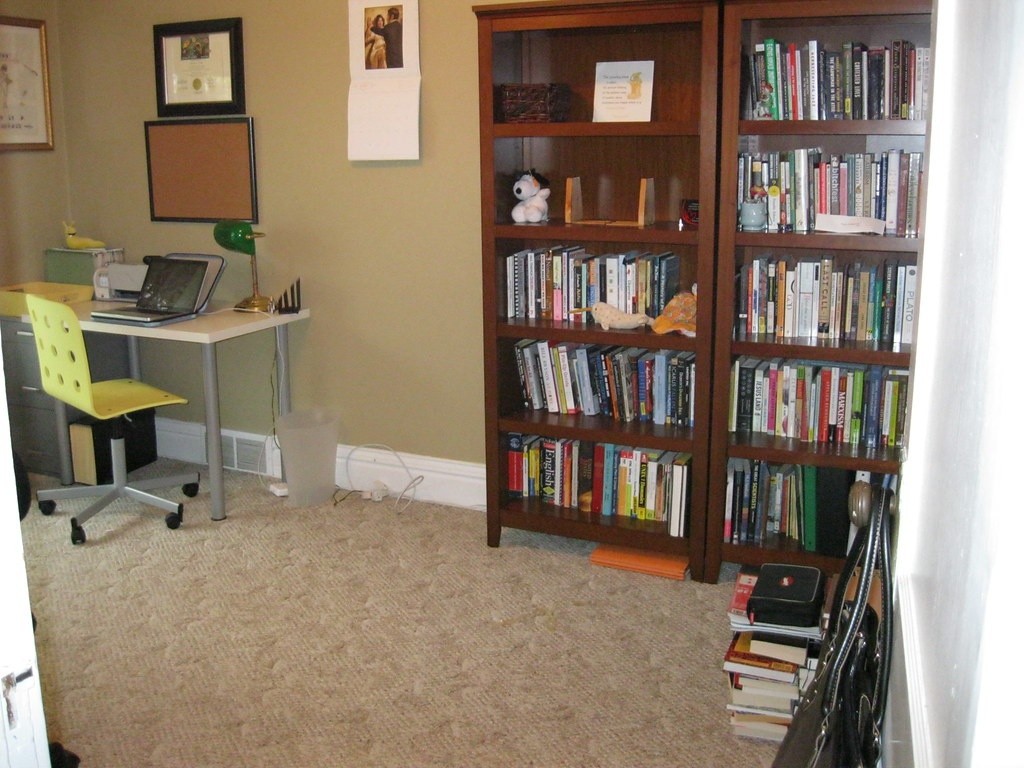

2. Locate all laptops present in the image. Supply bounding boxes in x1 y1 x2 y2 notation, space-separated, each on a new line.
90 258 210 322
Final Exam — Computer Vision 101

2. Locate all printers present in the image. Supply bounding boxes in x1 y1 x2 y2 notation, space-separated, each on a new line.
92 262 149 302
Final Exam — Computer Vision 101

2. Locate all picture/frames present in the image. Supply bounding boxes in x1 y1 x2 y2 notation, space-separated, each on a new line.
143 116 259 225
0 14 56 153
153 17 249 118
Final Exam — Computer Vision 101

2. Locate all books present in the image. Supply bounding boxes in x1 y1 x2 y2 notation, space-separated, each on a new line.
737 147 922 237
514 339 695 428
751 35 931 121
721 563 831 742
738 252 917 345
505 245 680 325
723 459 898 556
507 434 691 538
729 356 909 450
589 540 687 583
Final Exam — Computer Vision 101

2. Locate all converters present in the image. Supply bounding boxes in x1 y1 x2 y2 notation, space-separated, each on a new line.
269 481 289 497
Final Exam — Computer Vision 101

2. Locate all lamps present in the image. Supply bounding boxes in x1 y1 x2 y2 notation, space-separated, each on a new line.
213 220 278 314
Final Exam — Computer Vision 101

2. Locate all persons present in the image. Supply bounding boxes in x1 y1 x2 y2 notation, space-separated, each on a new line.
759 83 774 117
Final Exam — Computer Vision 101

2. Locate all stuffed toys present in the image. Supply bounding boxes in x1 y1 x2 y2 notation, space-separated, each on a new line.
510 169 550 223
567 283 697 337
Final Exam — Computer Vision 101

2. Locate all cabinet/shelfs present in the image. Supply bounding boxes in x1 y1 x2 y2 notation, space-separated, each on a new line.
0 301 312 520
473 0 940 587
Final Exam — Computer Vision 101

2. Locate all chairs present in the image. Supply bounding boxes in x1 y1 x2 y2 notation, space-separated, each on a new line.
26 294 201 546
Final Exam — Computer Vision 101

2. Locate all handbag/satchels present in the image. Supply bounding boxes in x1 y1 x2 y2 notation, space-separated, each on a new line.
768 484 893 768
747 562 827 627
